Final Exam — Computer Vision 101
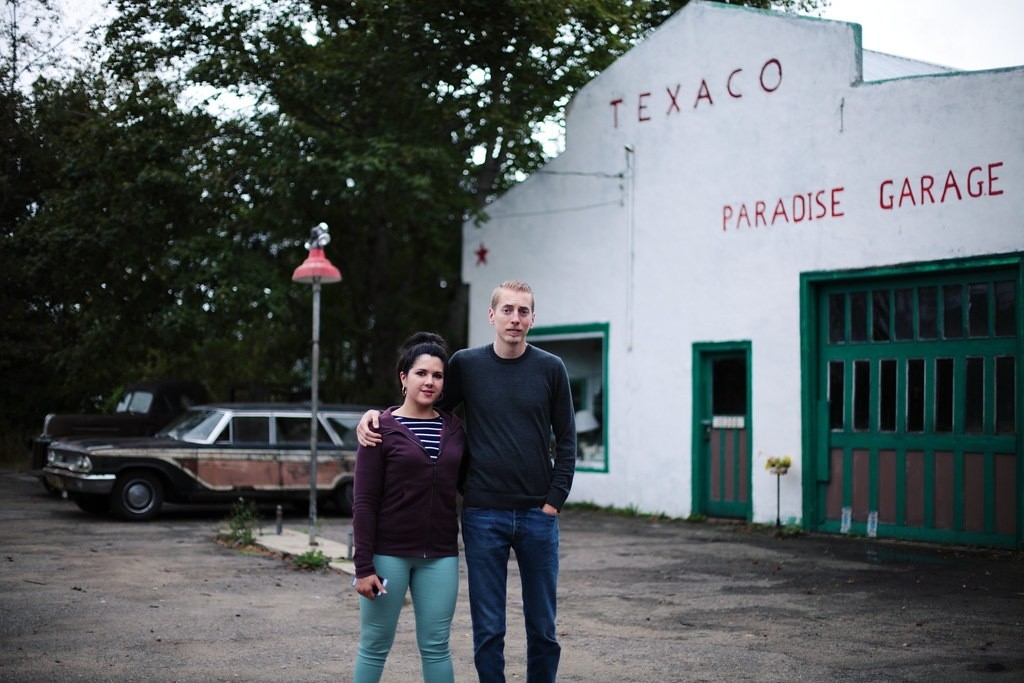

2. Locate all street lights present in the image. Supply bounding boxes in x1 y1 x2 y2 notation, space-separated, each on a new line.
293 222 341 546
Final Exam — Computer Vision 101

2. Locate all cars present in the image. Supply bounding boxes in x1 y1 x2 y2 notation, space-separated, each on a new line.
44 403 366 520
32 381 210 476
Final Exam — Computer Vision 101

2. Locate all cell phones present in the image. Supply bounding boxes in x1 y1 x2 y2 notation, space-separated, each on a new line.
351 574 388 598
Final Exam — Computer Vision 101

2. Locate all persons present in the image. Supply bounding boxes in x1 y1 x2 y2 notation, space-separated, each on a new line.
351 332 469 683
355 278 578 683
